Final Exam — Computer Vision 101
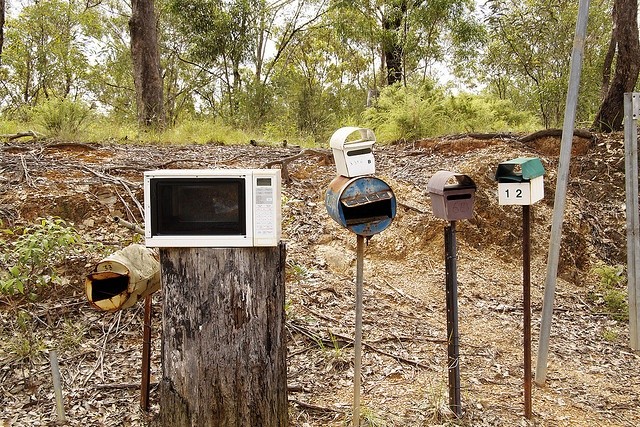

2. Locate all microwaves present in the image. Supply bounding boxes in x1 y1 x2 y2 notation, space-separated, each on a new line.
145 169 282 248
